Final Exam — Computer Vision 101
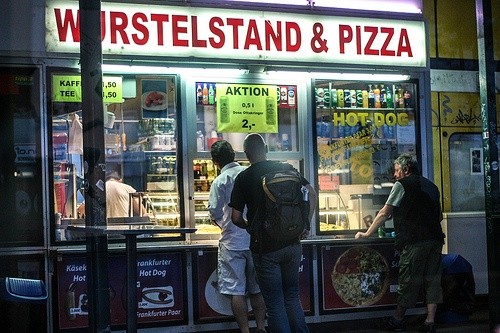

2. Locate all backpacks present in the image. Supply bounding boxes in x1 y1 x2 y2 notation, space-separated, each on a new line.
259 162 311 248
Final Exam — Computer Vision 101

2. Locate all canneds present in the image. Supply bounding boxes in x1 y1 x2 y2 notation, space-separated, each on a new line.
315 88 368 108
136 118 176 151
378 222 386 238
277 87 295 106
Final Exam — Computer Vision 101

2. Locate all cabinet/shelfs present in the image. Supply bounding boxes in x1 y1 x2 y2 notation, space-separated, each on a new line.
126 150 299 237
315 85 415 149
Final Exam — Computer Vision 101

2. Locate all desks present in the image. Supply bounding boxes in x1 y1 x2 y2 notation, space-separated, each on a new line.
67 223 197 333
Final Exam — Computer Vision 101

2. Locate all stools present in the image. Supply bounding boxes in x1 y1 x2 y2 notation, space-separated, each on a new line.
2 277 49 333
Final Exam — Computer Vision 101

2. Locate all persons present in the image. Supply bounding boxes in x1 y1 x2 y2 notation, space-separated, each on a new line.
207 141 269 333
355 153 445 333
229 133 316 332
78 169 146 218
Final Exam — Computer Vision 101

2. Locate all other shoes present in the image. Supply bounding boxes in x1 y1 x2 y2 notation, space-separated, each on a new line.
416 322 435 333
376 316 407 331
256 329 267 333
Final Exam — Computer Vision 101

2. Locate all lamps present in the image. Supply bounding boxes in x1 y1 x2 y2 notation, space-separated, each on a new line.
78 59 251 79
264 66 414 83
320 209 355 215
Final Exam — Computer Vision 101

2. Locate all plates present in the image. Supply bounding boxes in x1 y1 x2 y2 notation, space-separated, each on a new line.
142 91 168 111
205 268 254 315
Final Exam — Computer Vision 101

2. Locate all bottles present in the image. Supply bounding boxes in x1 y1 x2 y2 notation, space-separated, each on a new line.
277 87 295 107
208 84 215 105
314 83 414 109
145 154 182 226
138 119 176 151
193 160 216 228
203 83 209 105
196 83 203 104
378 222 385 238
197 129 224 152
